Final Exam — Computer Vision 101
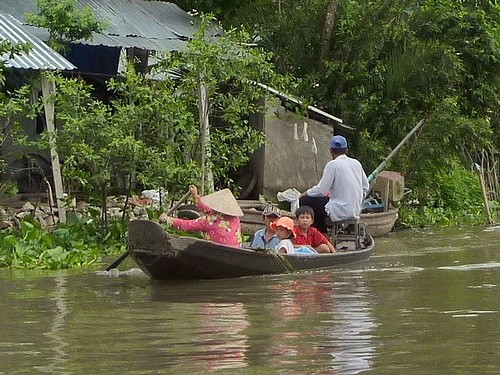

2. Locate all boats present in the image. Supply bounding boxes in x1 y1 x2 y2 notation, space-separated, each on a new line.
126 217 376 281
234 169 411 238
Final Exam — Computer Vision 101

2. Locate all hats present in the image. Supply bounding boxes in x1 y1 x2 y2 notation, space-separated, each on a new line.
270 217 296 238
331 135 347 148
262 205 282 217
200 189 244 217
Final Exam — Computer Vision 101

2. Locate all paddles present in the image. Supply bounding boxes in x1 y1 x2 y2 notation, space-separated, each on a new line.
105 190 191 271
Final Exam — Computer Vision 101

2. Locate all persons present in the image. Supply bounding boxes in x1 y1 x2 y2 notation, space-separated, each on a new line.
299 135 370 235
157 185 244 248
251 206 336 255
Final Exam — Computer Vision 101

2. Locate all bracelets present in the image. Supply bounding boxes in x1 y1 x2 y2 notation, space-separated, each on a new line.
163 215 167 222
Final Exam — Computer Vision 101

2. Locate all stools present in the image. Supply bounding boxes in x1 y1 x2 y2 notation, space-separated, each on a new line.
331 217 360 251
